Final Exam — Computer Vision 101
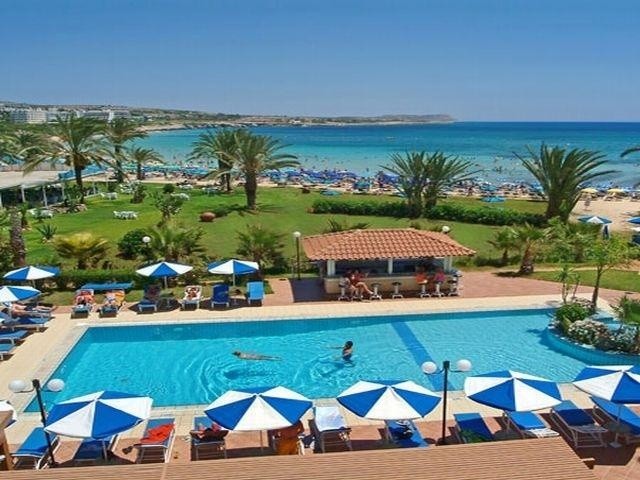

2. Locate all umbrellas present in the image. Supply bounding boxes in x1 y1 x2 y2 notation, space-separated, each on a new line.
45 387 154 459
0 284 41 322
626 216 640 226
207 258 259 302
2 264 60 289
203 384 314 454
135 260 193 305
576 213 613 228
572 362 640 446
464 368 564 433
338 377 443 441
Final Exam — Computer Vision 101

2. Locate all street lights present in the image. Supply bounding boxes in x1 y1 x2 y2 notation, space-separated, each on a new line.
293 231 301 280
142 236 150 266
422 360 471 445
8 379 65 468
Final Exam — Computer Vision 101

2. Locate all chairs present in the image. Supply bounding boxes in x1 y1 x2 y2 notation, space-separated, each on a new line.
309 406 353 452
550 400 609 449
384 417 429 447
453 413 498 443
135 417 177 462
0 304 53 359
72 434 118 464
72 282 264 317
192 415 227 460
590 395 640 445
502 411 560 439
267 428 306 453
11 426 62 469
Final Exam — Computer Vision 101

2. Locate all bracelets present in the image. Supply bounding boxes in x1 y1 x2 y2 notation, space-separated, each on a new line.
275 435 279 438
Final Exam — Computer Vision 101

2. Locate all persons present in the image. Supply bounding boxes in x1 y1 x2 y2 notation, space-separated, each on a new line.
346 274 375 296
100 290 120 309
340 340 353 359
603 224 609 236
431 267 445 283
232 351 280 362
189 420 229 444
185 286 199 301
10 302 57 312
147 284 161 303
350 270 366 299
417 266 429 285
2 307 55 321
272 417 305 456
71 288 96 310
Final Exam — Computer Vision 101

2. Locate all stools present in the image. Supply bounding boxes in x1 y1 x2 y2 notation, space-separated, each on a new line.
338 279 460 300
